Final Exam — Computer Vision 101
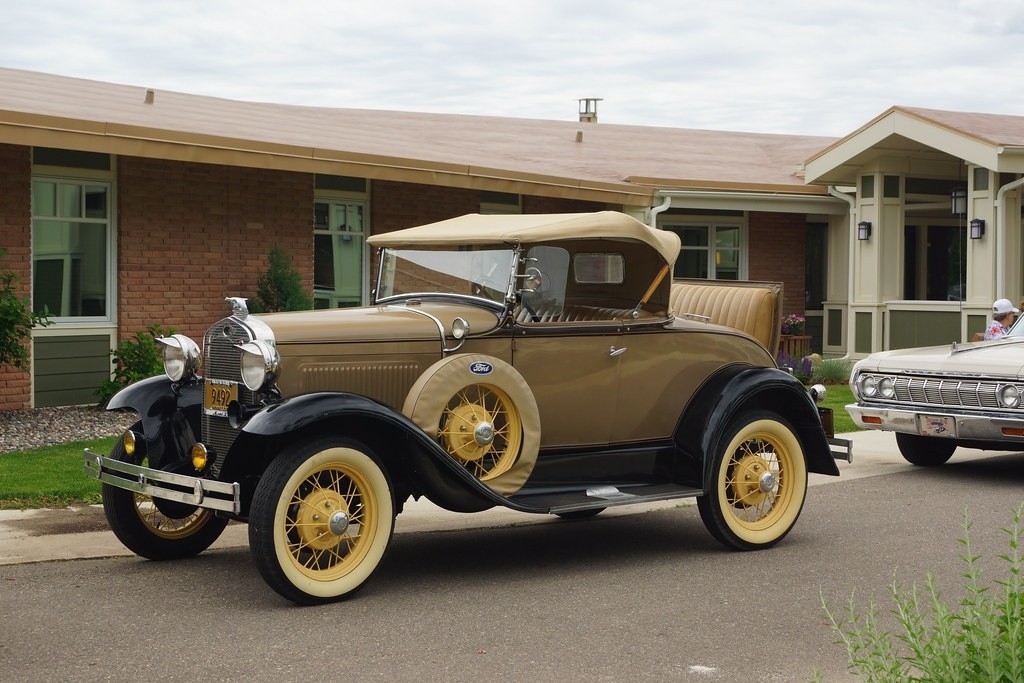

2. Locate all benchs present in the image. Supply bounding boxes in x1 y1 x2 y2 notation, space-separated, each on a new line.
510 301 653 323
671 282 775 353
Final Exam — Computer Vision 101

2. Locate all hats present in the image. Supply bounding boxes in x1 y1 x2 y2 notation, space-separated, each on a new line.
994 299 1019 314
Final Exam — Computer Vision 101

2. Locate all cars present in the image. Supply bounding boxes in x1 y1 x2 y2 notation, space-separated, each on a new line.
842 303 1024 465
84 211 858 606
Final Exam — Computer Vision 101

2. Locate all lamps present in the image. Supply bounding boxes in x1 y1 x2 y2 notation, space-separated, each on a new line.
970 219 985 239
952 158 966 215
858 221 871 239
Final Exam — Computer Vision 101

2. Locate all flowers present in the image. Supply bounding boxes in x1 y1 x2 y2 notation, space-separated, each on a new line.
781 314 806 336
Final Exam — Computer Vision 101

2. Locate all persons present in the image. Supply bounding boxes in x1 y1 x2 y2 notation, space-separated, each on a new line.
984 299 1019 341
